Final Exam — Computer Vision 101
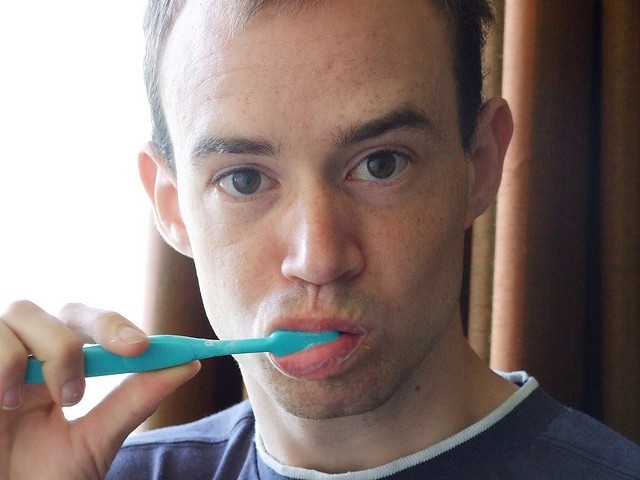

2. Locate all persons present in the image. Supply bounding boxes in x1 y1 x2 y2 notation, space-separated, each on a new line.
1 0 640 360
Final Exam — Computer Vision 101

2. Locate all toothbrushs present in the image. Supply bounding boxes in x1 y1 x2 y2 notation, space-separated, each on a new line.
24 330 341 385
